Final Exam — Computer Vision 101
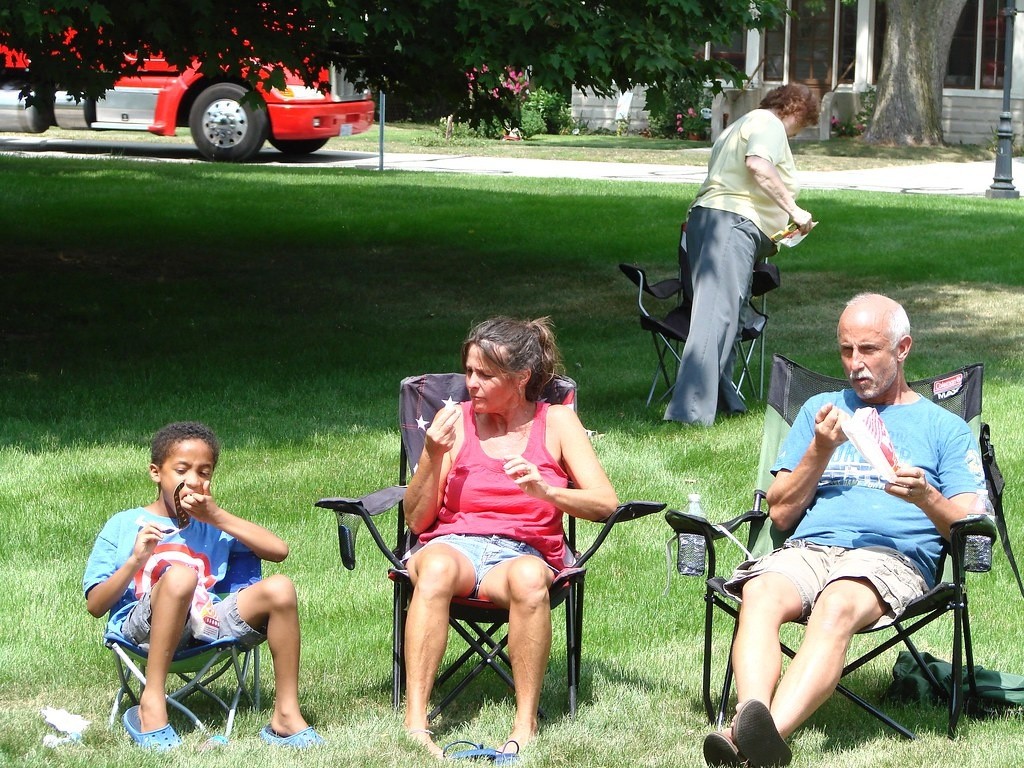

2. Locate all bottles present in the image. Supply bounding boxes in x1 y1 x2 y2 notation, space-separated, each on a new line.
963 490 995 573
677 494 706 577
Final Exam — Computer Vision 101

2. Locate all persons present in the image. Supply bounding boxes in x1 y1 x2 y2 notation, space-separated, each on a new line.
664 84 819 427
403 316 617 758
84 422 326 752
703 293 987 768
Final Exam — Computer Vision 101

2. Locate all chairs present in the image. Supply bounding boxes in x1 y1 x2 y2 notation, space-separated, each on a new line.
314 374 668 722
665 353 1024 742
104 553 262 746
620 223 781 406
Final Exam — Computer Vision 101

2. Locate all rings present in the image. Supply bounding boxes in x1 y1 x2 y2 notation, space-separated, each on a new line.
907 488 913 498
527 466 531 473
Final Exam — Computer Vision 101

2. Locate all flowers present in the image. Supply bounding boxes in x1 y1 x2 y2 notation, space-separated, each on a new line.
676 106 711 135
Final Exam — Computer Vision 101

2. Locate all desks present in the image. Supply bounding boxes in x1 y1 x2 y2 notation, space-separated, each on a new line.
699 52 745 69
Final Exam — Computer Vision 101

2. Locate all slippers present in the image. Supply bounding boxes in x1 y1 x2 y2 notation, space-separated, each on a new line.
494 741 520 766
442 741 498 761
259 723 326 749
122 705 183 752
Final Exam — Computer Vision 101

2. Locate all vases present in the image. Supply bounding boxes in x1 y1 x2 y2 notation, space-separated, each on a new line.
690 132 698 140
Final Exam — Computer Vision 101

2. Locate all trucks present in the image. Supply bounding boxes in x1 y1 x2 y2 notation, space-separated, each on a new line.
0 0 378 163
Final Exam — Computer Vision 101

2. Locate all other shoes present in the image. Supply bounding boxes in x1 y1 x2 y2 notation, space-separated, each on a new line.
702 733 739 768
731 699 792 768
